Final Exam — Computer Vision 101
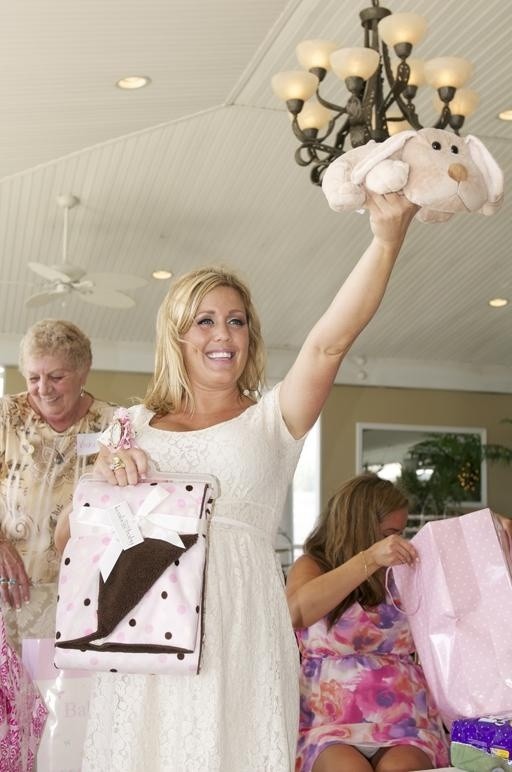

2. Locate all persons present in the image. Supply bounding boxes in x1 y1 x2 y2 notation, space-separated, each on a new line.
285 468 511 772
36 183 422 772
0 318 123 771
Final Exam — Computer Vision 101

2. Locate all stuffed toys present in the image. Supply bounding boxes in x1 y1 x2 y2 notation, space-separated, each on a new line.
322 126 506 228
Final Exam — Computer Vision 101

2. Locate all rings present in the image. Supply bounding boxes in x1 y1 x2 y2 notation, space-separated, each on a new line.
1 575 9 584
9 577 17 585
109 457 125 473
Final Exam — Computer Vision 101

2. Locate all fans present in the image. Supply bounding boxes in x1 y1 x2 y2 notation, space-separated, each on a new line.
24 189 137 315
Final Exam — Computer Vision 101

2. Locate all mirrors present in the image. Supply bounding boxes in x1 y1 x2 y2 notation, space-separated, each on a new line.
352 418 488 521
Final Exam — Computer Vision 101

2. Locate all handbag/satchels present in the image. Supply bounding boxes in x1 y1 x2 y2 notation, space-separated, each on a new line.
380 504 512 725
47 467 222 680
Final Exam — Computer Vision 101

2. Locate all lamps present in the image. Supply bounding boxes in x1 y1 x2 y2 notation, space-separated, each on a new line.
266 1 486 188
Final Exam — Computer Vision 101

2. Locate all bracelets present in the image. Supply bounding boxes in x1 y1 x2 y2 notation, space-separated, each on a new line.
357 549 372 582
